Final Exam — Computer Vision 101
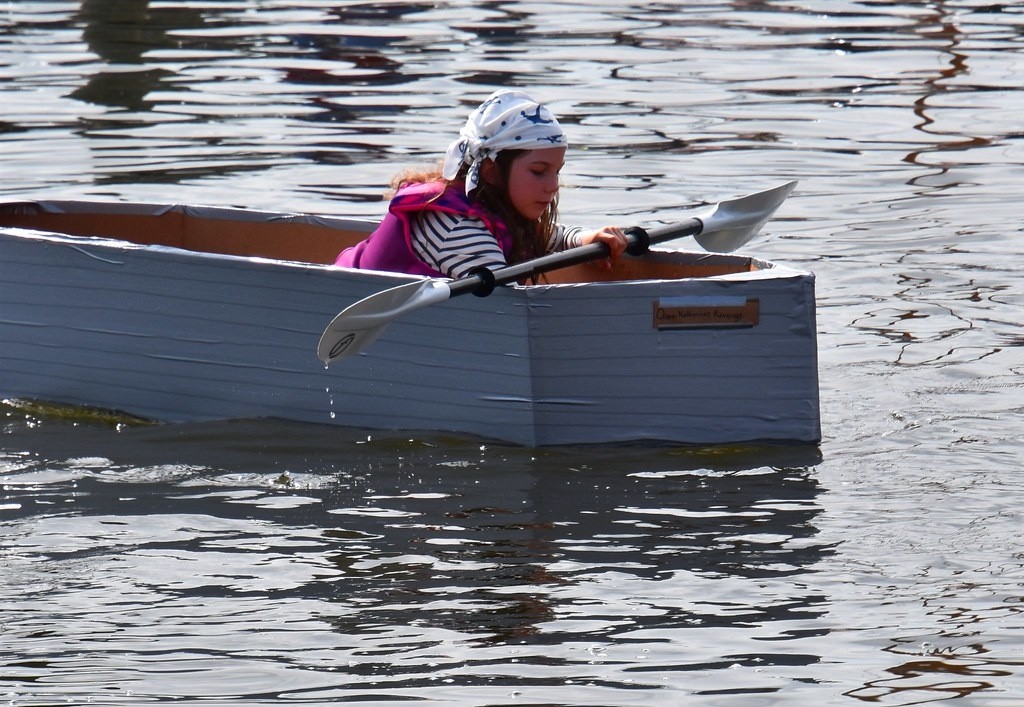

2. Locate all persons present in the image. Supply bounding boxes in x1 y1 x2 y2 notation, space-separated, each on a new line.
335 88 628 286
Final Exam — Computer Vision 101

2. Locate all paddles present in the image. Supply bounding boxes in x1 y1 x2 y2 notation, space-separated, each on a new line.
315 176 802 367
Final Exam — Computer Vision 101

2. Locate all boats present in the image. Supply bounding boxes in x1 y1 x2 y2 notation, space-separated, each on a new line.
0 193 822 447
